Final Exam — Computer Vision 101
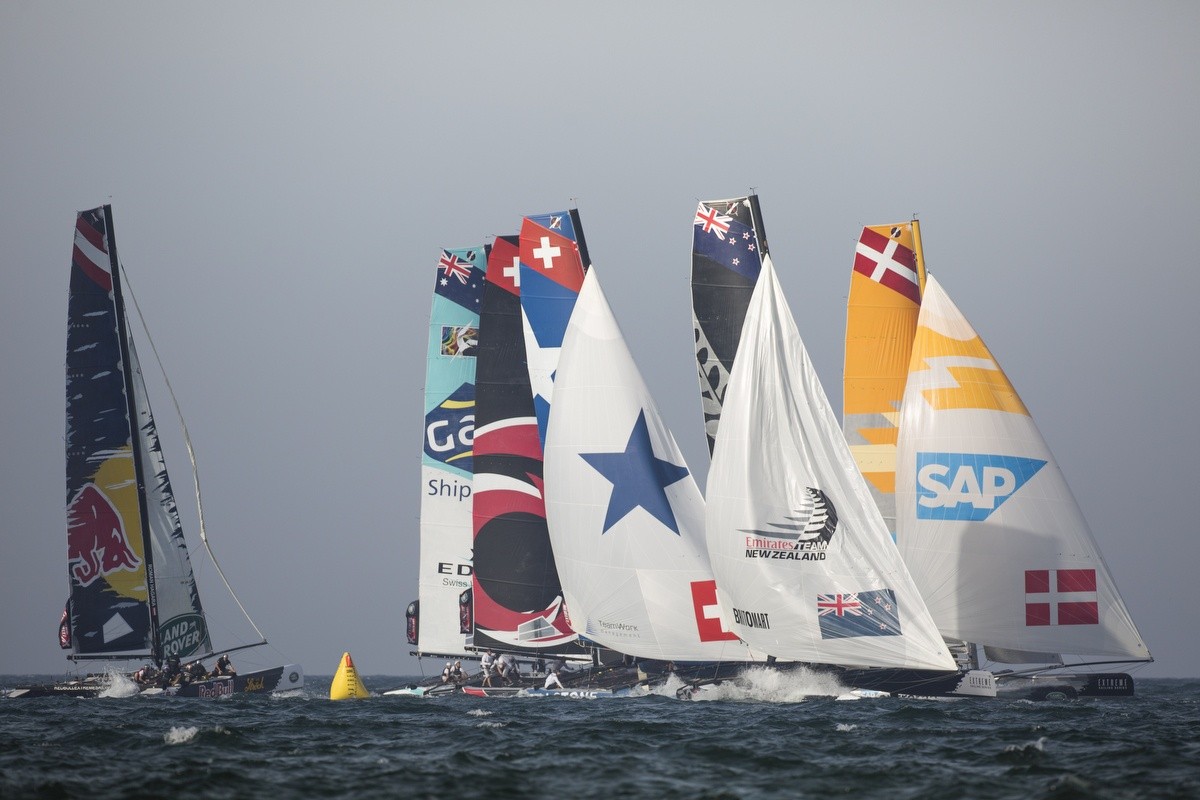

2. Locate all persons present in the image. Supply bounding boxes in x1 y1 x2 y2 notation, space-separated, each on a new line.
442 660 467 683
135 654 236 688
481 649 519 688
544 670 564 690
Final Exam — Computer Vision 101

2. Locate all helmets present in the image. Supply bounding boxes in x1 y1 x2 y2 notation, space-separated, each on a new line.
223 654 228 661
144 664 149 670
197 659 200 664
162 664 170 672
494 653 499 658
174 652 178 656
455 660 460 664
186 664 193 671
446 662 451 666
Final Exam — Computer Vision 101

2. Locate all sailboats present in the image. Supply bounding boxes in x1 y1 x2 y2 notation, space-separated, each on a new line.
381 190 1151 699
58 204 304 703
